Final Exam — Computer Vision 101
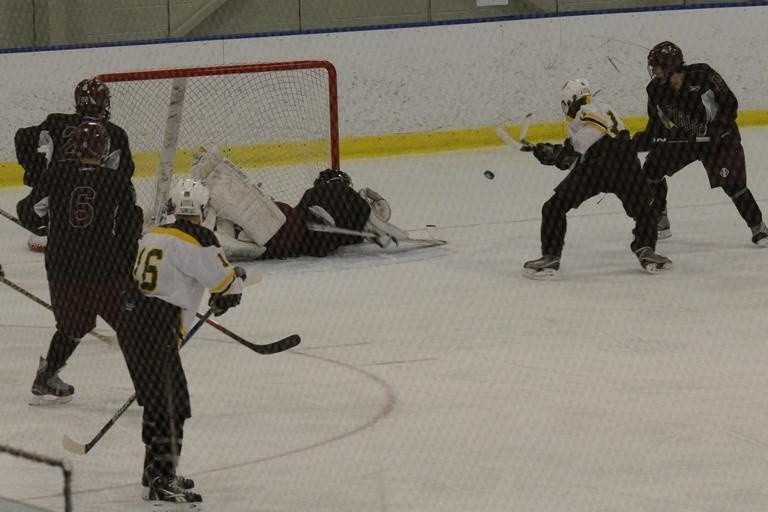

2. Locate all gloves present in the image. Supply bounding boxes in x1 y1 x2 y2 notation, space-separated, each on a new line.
706 119 733 155
209 293 242 315
633 131 654 151
532 141 562 165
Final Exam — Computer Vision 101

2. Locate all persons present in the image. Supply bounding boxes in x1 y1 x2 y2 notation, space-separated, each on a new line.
524 76 673 271
630 39 768 248
16 115 141 397
188 144 372 261
14 78 137 187
114 176 245 504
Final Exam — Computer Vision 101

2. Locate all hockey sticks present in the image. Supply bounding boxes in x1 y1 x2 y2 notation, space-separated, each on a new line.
63 308 213 455
0 208 302 354
496 113 711 152
306 224 447 245
0 276 119 346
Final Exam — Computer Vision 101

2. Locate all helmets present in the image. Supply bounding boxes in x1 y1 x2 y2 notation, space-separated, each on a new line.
314 169 353 188
169 178 210 223
75 79 110 119
74 122 110 164
561 79 590 118
647 41 684 85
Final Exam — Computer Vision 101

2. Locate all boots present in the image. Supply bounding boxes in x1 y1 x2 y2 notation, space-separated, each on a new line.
524 254 560 271
31 357 75 396
635 246 672 268
632 200 670 234
750 221 768 244
143 446 203 504
